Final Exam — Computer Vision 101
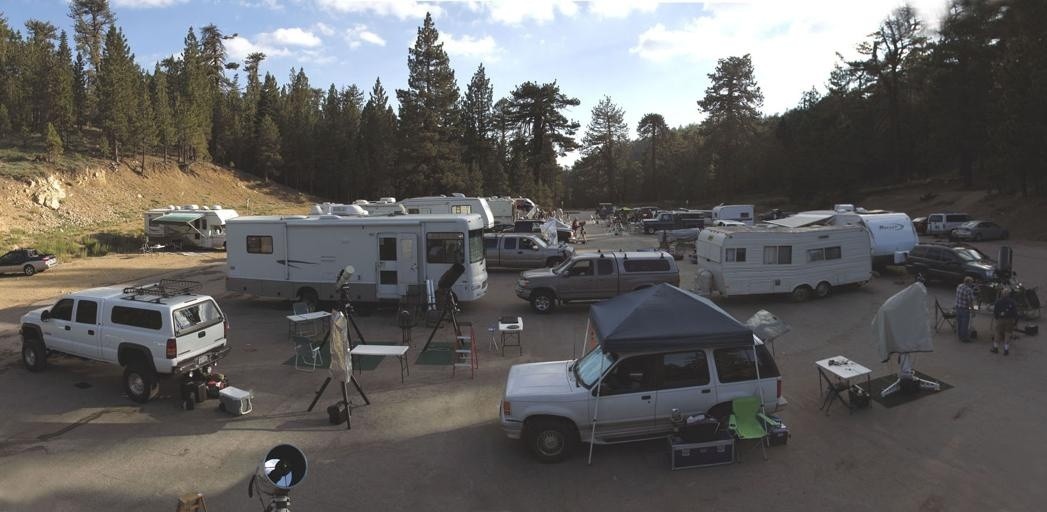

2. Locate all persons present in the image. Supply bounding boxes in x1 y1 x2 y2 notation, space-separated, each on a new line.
989 287 1014 355
516 204 654 244
954 276 978 342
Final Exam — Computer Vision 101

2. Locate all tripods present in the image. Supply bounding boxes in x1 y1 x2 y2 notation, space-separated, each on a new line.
422 304 465 358
320 300 366 353
308 373 370 430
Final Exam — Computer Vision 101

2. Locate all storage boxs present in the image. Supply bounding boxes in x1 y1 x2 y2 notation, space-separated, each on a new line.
219 386 251 416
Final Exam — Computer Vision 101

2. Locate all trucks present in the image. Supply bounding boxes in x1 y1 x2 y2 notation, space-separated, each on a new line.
310 195 538 232
711 204 753 226
699 221 872 301
833 205 917 271
598 202 704 235
227 213 488 306
143 205 238 251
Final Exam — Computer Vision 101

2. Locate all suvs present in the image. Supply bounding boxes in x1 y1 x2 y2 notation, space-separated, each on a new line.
497 327 786 463
904 242 1004 287
512 221 573 242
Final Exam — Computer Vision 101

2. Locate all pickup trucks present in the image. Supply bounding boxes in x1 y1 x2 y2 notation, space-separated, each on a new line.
0 248 57 276
483 232 574 271
19 277 229 403
511 248 680 314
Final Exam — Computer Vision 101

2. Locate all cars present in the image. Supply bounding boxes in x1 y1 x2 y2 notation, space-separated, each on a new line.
953 220 1008 241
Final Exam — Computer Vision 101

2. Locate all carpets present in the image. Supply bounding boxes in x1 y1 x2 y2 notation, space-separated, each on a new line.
282 341 397 370
415 341 473 365
856 368 955 409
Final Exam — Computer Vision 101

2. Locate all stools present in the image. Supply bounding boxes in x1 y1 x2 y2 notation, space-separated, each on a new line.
179 496 207 512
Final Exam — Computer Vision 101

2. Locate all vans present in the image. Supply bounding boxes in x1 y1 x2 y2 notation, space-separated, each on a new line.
928 213 970 235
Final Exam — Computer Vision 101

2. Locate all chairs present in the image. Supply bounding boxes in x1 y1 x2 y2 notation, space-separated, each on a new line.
934 296 957 334
728 395 770 464
819 368 853 413
293 337 324 373
291 303 318 338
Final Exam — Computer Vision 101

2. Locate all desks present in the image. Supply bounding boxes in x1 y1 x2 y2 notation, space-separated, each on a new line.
350 344 410 385
499 316 524 357
967 305 1039 341
286 310 331 337
815 354 873 415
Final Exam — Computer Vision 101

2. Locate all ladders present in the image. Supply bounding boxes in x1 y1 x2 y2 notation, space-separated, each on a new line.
452 322 479 380
176 494 207 512
